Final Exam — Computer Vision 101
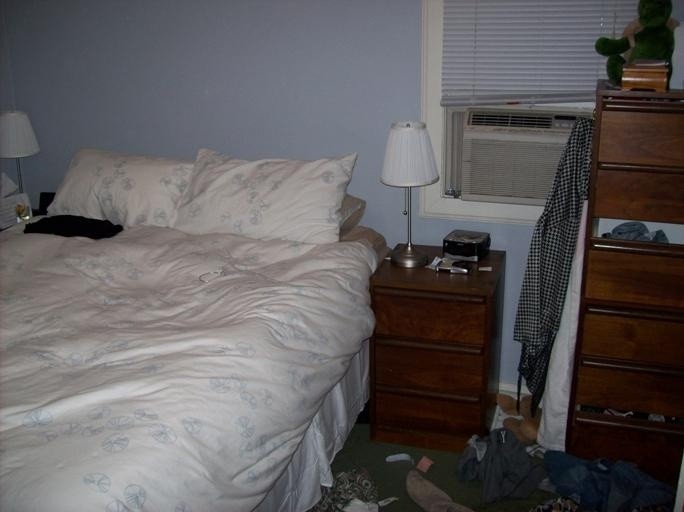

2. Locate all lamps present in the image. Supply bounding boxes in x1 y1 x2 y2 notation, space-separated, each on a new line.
380 120 438 269
1 109 44 224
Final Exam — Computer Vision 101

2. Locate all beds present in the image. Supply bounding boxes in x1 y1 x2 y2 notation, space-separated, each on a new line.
0 149 391 512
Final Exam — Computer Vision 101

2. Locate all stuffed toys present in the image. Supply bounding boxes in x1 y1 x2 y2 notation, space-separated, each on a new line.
595 0 676 91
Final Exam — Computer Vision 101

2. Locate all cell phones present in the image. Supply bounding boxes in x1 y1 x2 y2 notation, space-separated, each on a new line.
436 259 478 275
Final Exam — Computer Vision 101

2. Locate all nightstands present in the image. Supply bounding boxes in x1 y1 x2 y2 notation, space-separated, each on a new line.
368 242 506 452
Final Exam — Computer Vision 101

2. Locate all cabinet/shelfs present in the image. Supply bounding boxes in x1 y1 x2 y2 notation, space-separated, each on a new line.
564 77 684 487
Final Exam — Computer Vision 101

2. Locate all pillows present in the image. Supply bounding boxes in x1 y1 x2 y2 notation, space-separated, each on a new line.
47 151 193 229
172 149 358 242
